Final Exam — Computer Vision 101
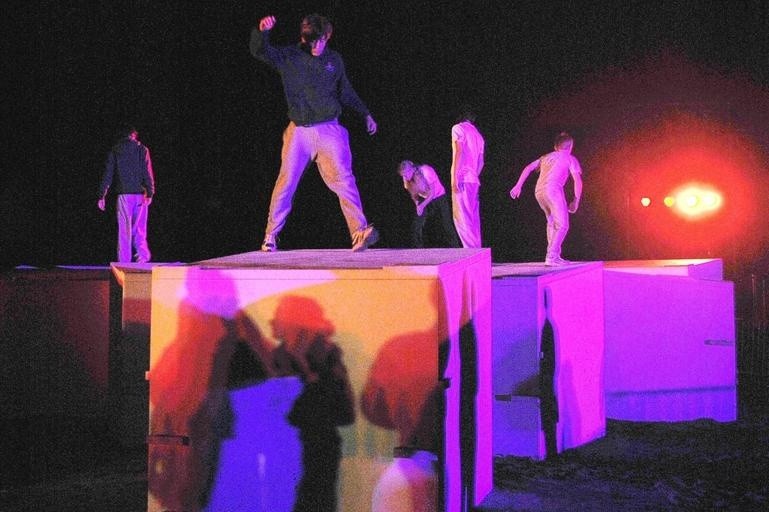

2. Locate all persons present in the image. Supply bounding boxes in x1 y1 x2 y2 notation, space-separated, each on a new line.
98 124 155 265
245 13 383 253
395 104 487 248
508 132 583 266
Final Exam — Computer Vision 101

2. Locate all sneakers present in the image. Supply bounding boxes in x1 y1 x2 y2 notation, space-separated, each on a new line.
350 225 382 253
543 257 571 268
262 234 281 252
